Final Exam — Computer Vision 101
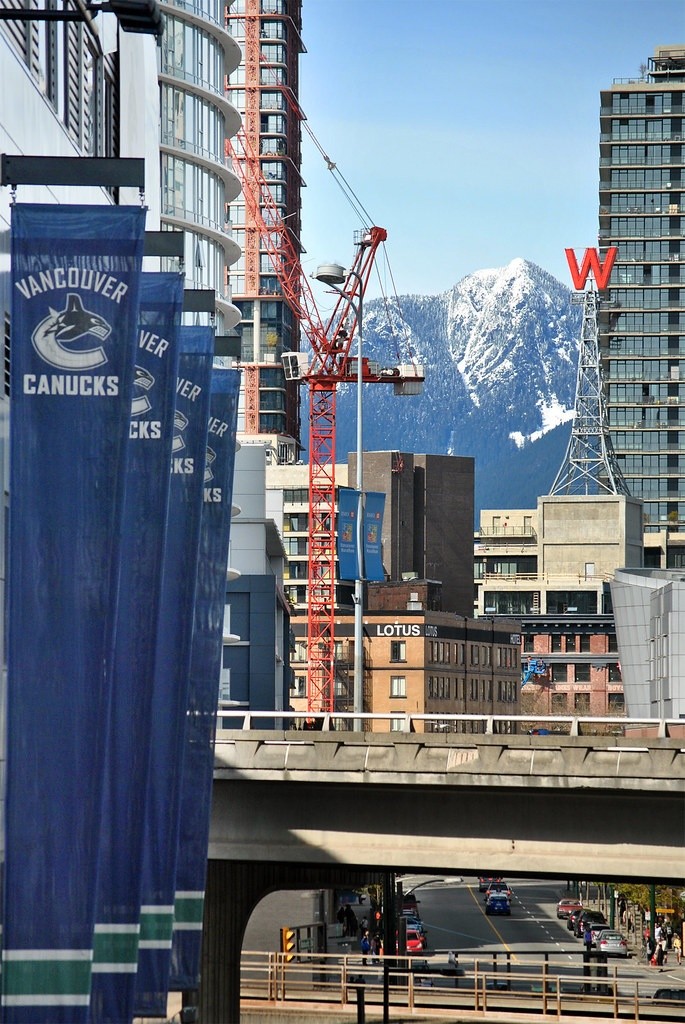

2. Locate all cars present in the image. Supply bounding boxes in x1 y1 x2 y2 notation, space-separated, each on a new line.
483 893 511 915
485 881 512 901
393 888 428 955
478 877 502 892
556 898 628 958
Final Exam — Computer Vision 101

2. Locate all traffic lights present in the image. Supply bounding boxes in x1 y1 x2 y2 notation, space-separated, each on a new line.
374 911 381 921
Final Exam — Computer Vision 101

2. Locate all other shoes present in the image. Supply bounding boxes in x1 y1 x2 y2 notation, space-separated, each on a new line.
679 963 681 965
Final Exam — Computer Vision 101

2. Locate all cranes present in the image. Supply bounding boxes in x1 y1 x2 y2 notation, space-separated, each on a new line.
228 123 425 730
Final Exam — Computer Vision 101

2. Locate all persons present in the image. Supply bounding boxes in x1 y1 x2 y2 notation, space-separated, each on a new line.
618 899 683 972
336 903 382 965
348 975 367 988
